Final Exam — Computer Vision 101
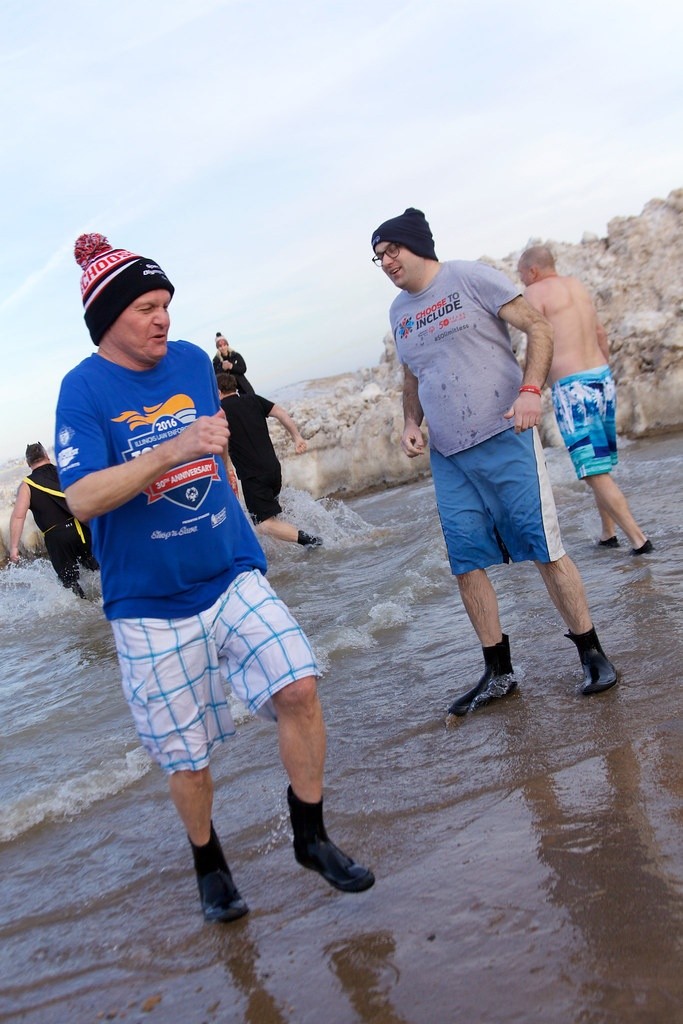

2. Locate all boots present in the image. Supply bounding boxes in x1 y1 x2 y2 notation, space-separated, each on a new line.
285 786 375 892
184 820 249 921
447 633 518 716
565 623 618 695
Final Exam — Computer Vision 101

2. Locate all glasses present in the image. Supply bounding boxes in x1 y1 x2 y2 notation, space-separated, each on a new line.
372 241 404 267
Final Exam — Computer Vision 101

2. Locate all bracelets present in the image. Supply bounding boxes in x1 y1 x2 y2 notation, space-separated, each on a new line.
519 385 542 397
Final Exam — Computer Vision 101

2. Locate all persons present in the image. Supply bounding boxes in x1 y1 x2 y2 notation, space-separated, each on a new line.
55 232 375 925
212 332 256 396
9 441 101 600
371 207 619 718
515 246 655 557
216 372 323 552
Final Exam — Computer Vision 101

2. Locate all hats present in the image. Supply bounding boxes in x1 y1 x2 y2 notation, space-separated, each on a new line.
216 332 228 347
371 207 437 260
74 233 174 346
